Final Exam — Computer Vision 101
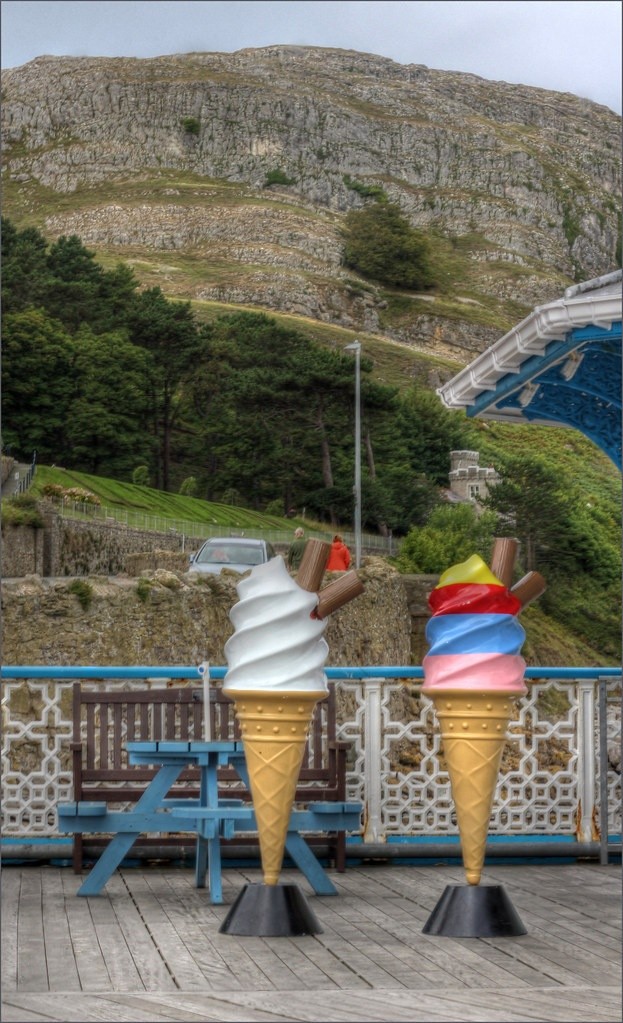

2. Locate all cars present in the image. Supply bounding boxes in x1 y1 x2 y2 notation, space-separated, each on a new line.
187 537 277 575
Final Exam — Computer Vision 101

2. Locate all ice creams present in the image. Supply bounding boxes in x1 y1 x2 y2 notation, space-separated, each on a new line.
421 555 528 886
222 555 328 886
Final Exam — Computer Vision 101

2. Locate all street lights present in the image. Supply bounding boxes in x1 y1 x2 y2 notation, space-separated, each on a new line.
343 340 364 570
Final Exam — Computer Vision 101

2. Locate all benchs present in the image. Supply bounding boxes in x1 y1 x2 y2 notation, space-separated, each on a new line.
70 680 351 873
57 800 363 834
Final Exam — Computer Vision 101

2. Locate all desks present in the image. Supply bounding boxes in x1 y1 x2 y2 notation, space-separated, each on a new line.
77 741 339 906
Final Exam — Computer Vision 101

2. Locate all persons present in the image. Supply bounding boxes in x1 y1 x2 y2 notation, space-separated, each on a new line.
325 535 350 571
288 526 308 572
